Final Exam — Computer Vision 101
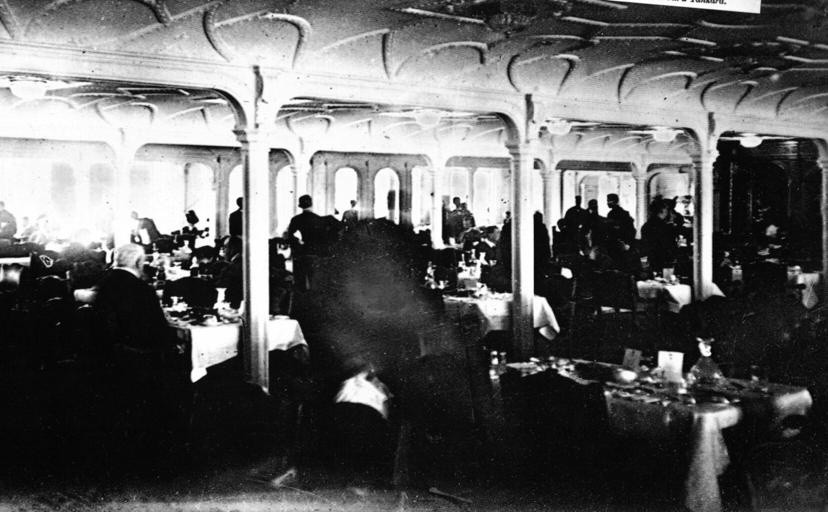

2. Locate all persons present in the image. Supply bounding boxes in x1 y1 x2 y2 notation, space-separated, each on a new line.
283 193 691 289
2 197 245 399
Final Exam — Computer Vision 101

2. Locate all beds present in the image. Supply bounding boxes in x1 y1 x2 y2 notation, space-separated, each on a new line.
438 267 820 511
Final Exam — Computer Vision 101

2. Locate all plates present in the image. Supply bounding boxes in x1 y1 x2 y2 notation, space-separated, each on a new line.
507 361 539 375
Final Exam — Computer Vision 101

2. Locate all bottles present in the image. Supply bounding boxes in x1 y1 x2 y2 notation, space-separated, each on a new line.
688 335 726 384
487 350 506 377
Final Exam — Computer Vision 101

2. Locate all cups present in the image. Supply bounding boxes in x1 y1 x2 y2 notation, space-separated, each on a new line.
662 268 675 279
